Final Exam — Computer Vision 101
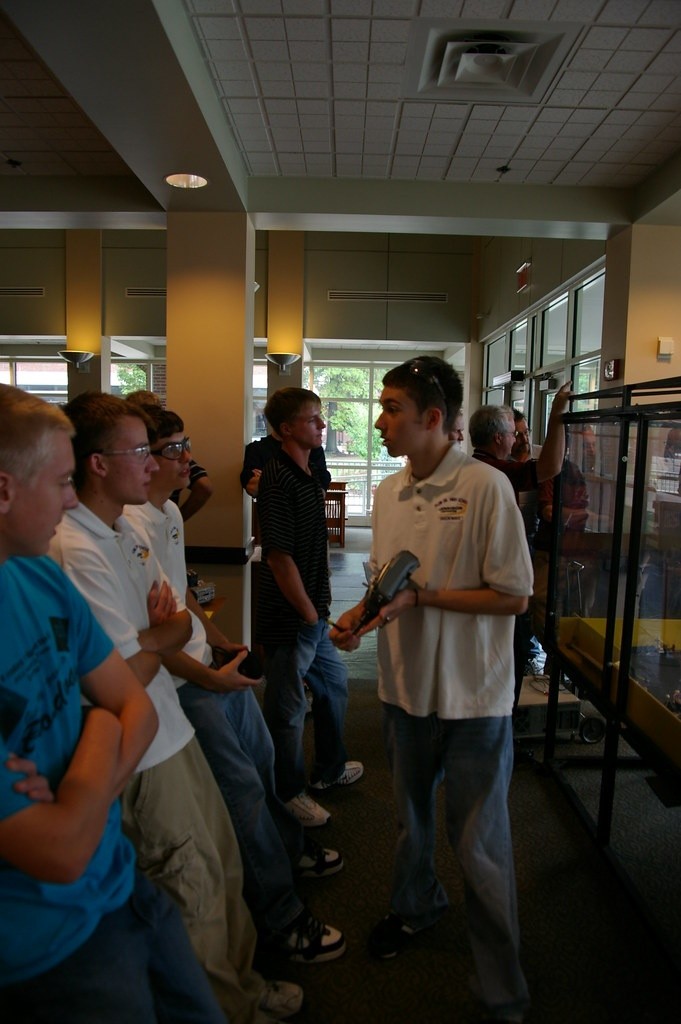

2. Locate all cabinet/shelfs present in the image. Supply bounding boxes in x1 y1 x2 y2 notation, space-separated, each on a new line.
536 375 681 995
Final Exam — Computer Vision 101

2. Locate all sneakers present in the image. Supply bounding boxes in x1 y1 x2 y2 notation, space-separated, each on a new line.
363 903 451 963
293 845 343 879
283 792 331 827
310 760 364 789
273 915 346 964
257 980 303 1018
477 1002 524 1024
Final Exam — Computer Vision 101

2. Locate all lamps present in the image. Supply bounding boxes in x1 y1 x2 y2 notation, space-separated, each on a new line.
57 350 94 374
265 352 302 376
538 378 557 391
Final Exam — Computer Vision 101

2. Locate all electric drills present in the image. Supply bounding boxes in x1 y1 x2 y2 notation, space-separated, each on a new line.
351 549 423 636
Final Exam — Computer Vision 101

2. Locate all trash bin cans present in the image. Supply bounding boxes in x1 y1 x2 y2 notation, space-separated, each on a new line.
324 481 348 548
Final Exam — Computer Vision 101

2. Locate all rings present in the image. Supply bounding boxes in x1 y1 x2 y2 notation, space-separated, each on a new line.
387 617 390 623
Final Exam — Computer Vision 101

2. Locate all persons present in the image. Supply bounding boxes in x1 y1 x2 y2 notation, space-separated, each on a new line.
658 428 681 494
580 425 595 473
327 356 589 1024
0 388 363 1024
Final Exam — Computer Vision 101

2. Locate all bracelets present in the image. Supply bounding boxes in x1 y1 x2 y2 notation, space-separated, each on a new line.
412 588 419 608
207 630 226 647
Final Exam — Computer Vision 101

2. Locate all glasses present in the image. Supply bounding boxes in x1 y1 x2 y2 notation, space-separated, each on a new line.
407 357 445 409
147 436 191 460
504 431 518 440
100 444 150 465
516 429 533 439
